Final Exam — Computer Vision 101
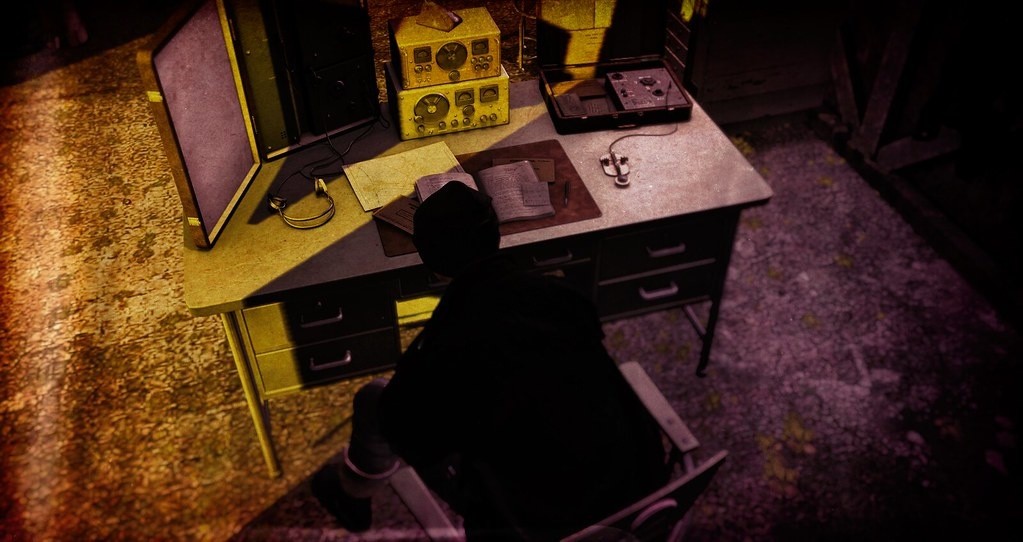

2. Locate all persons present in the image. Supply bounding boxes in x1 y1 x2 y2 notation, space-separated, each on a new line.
311 181 665 541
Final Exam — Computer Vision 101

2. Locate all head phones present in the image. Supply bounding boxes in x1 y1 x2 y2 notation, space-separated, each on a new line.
267 177 335 230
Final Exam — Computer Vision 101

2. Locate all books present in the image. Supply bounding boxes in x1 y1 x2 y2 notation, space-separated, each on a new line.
414 160 555 225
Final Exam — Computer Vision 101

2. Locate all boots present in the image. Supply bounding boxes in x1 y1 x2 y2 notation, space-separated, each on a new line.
305 448 400 533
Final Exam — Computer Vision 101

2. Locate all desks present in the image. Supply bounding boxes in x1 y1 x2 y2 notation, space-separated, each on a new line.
184 80 774 482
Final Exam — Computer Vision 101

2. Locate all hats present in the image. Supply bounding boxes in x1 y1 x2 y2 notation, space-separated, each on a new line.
411 181 501 273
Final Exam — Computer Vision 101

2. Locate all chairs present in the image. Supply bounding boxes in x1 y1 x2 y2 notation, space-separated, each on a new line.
388 362 729 542
